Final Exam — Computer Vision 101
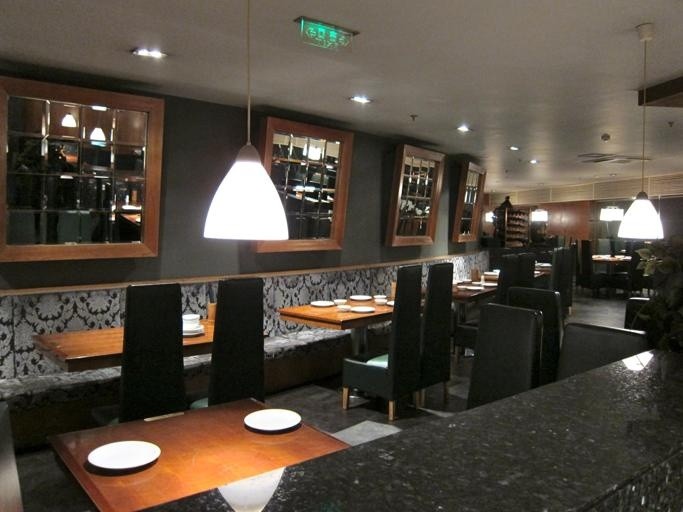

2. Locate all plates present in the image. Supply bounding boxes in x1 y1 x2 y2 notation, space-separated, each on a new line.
591 254 632 260
87 440 163 470
182 328 204 336
310 301 334 307
452 278 498 290
334 294 395 313
483 269 500 276
534 261 553 274
243 408 304 432
135 217 140 223
121 204 136 210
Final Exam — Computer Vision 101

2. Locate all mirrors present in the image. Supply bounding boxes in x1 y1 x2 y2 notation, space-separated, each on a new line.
385 144 446 247
0 76 165 261
250 115 355 252
450 159 485 243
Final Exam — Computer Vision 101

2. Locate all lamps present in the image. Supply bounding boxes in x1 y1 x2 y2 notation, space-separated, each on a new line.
86 108 106 141
60 104 77 127
599 174 624 222
295 137 324 161
619 22 666 240
202 1 290 240
529 183 549 222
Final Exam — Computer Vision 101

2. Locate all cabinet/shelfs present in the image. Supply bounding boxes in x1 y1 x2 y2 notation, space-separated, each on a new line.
496 209 531 250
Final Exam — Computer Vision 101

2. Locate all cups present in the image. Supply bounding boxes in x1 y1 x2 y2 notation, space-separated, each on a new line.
183 313 200 329
